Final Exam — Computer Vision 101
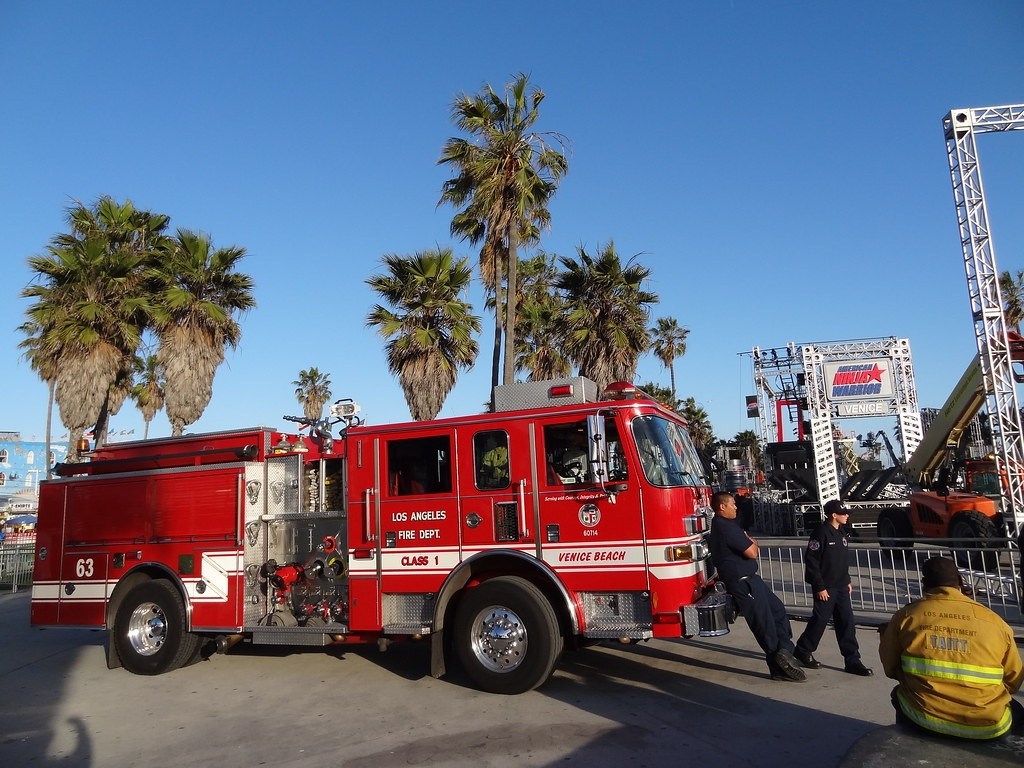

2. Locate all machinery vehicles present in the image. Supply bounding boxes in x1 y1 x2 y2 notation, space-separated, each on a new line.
876 328 1024 569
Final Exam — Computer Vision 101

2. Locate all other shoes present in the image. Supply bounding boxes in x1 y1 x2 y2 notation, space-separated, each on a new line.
775 649 808 681
771 674 794 682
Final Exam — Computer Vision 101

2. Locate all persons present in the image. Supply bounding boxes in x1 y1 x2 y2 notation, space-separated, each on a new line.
706 490 808 683
879 557 1024 742
794 500 872 676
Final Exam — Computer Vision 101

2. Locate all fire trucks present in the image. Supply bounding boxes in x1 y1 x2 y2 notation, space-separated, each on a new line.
31 376 739 694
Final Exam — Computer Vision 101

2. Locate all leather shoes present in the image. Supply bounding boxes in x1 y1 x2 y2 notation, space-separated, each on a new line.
846 660 873 676
793 646 823 669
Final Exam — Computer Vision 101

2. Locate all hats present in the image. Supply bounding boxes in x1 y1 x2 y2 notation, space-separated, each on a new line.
824 500 853 514
